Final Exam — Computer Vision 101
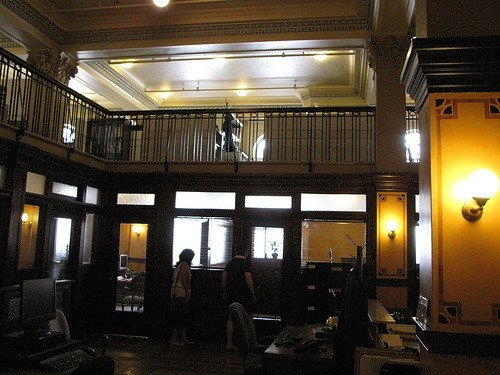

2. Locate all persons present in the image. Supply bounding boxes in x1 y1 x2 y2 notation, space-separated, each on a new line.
221 243 256 352
168 249 195 346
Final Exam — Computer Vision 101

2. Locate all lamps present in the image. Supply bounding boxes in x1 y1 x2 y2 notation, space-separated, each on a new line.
461 168 499 223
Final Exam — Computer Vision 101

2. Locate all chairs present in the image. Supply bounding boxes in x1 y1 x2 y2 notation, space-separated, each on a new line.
229 302 278 375
116 270 147 312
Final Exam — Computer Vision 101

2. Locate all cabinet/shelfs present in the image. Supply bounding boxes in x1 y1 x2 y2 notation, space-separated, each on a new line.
303 261 351 342
84 118 133 162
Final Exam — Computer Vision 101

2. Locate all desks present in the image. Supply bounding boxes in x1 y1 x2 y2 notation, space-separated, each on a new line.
262 323 335 375
366 298 396 345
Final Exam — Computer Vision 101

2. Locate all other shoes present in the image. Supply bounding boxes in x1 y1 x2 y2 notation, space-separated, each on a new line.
226 347 240 352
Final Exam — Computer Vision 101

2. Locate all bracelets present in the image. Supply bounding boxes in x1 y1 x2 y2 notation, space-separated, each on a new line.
251 293 256 296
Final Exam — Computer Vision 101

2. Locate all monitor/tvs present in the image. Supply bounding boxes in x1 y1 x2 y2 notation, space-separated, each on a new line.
21 278 57 336
121 253 128 268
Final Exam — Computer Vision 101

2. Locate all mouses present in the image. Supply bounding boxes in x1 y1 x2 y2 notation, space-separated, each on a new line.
80 346 96 357
285 338 296 343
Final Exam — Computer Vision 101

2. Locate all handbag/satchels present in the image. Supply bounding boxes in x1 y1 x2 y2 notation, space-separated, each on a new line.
165 297 192 321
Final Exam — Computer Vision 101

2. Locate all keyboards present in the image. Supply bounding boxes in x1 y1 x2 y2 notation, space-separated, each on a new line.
287 325 305 339
40 350 92 372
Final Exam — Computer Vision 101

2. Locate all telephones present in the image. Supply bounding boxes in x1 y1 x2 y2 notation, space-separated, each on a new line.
295 340 323 352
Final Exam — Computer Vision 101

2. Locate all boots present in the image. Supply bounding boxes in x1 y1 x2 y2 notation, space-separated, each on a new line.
170 329 185 346
181 328 195 345
